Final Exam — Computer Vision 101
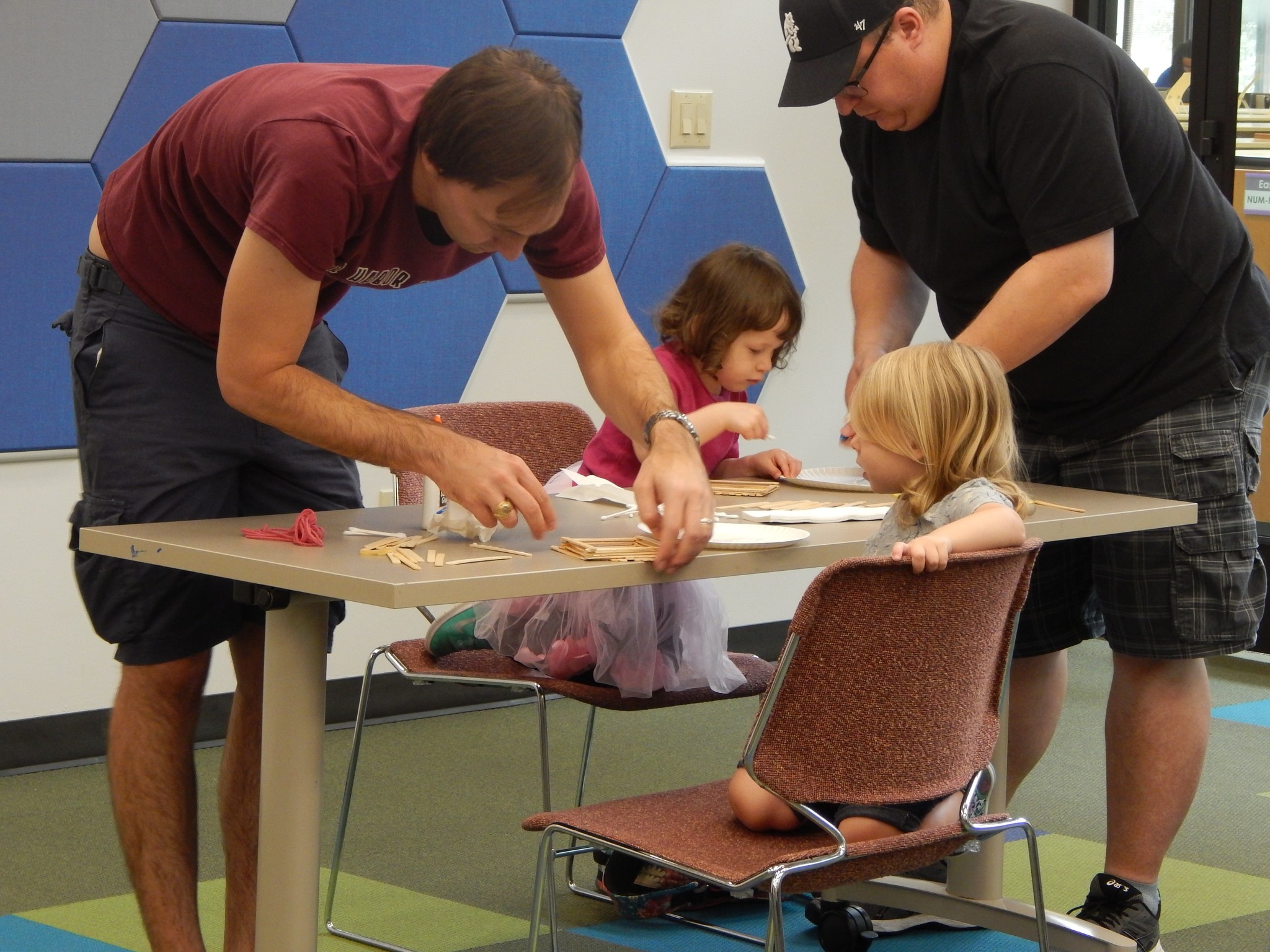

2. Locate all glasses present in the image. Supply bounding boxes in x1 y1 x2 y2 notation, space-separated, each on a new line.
843 21 891 102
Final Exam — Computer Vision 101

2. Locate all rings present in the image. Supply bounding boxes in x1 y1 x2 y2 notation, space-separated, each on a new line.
492 499 515 519
700 517 714 525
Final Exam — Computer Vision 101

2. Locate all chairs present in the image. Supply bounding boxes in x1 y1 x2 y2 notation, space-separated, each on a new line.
315 400 775 952
521 534 1050 952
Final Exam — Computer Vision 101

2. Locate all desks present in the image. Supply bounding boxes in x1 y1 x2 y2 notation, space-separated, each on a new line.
81 474 1198 952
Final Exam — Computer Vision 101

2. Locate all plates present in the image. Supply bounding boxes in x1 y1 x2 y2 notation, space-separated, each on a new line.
778 467 873 492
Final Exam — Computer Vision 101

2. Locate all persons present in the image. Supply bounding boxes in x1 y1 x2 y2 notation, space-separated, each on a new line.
777 1 1270 952
49 46 716 951
1155 39 1192 104
426 244 803 656
728 343 1032 841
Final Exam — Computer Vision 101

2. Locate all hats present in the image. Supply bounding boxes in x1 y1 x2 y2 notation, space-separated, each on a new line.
777 1 901 109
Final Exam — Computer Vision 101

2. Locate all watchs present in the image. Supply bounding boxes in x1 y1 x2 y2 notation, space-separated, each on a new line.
643 409 700 446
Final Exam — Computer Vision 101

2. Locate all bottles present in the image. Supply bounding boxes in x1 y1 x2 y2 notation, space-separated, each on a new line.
421 413 449 529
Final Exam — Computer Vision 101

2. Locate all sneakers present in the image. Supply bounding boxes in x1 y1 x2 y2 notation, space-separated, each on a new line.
1066 873 1165 952
806 860 978 933
